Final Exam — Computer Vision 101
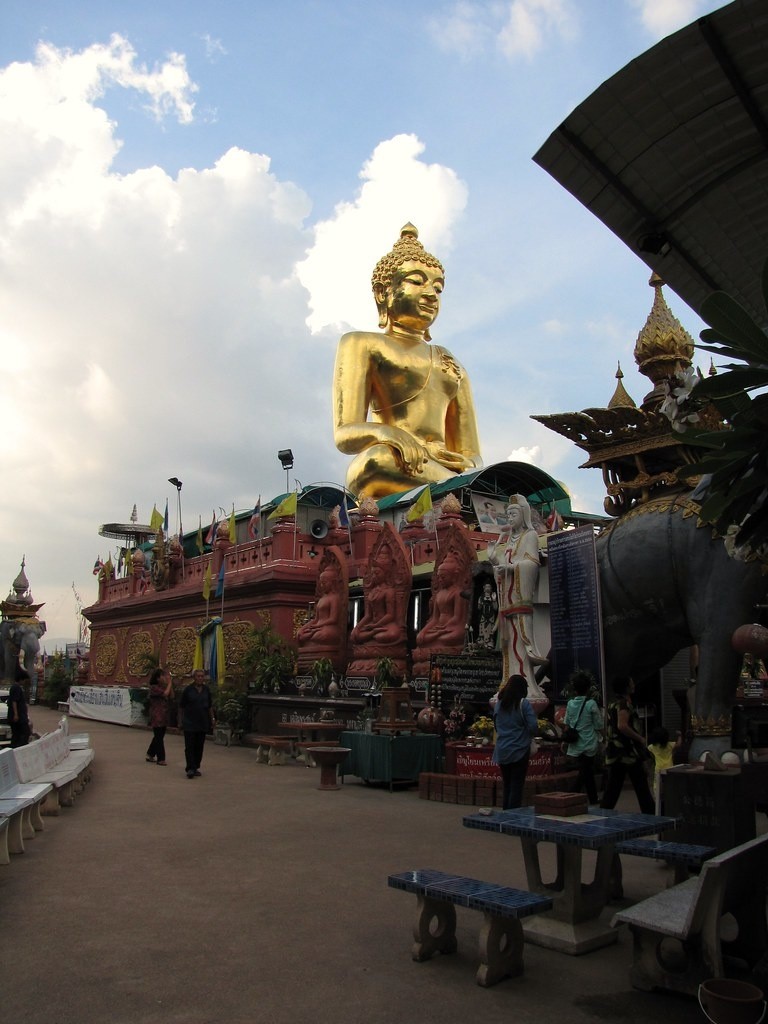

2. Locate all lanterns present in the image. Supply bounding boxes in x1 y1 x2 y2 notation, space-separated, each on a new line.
732 623 768 655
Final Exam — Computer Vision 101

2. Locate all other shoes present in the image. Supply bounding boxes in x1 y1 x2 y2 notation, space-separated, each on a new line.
185 767 201 776
187 770 194 778
157 761 167 765
145 756 156 762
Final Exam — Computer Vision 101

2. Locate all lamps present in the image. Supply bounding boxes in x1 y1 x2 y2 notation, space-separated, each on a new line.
168 478 183 540
277 449 294 496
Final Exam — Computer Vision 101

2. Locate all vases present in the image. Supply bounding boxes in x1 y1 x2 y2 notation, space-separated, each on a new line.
447 730 464 741
474 732 558 746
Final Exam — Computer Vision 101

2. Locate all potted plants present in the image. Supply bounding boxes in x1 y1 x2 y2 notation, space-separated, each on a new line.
372 657 404 687
211 687 245 745
310 657 335 696
238 619 296 694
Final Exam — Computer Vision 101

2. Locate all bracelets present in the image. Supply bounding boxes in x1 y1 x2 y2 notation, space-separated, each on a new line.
212 718 215 720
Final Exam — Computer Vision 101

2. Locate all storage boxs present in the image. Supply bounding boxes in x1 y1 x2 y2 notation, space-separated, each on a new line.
533 791 589 817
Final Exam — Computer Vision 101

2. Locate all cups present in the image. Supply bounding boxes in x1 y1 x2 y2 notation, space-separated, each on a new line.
473 736 475 746
534 737 542 747
466 737 472 746
476 738 483 747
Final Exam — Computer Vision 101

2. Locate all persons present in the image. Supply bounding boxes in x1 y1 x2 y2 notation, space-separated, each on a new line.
350 559 400 644
177 669 216 777
563 672 604 804
480 502 507 525
416 553 466 646
333 221 484 500
145 669 172 765
600 675 655 815
487 494 545 697
297 566 340 644
492 675 538 810
6 671 31 749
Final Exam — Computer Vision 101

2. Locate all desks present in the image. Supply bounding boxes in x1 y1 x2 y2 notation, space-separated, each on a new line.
306 746 350 791
462 808 685 955
338 730 445 793
450 744 563 777
278 721 347 765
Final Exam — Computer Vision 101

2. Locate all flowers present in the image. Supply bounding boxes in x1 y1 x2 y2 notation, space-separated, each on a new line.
444 705 548 737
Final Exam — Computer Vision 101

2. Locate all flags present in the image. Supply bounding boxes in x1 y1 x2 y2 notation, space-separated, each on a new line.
546 508 559 531
196 511 236 600
267 492 296 520
248 500 259 538
151 505 166 530
407 487 432 521
335 497 349 528
93 550 134 578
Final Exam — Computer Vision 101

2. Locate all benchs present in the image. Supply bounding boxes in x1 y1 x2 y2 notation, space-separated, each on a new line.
387 867 555 988
58 697 70 712
608 831 768 1008
0 715 95 865
611 838 717 888
251 735 338 767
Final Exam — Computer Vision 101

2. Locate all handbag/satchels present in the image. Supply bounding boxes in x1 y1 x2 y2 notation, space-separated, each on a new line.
561 728 580 743
529 739 537 757
639 749 655 777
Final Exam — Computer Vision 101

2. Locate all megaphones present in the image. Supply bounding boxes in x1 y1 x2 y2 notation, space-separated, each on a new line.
309 519 328 538
